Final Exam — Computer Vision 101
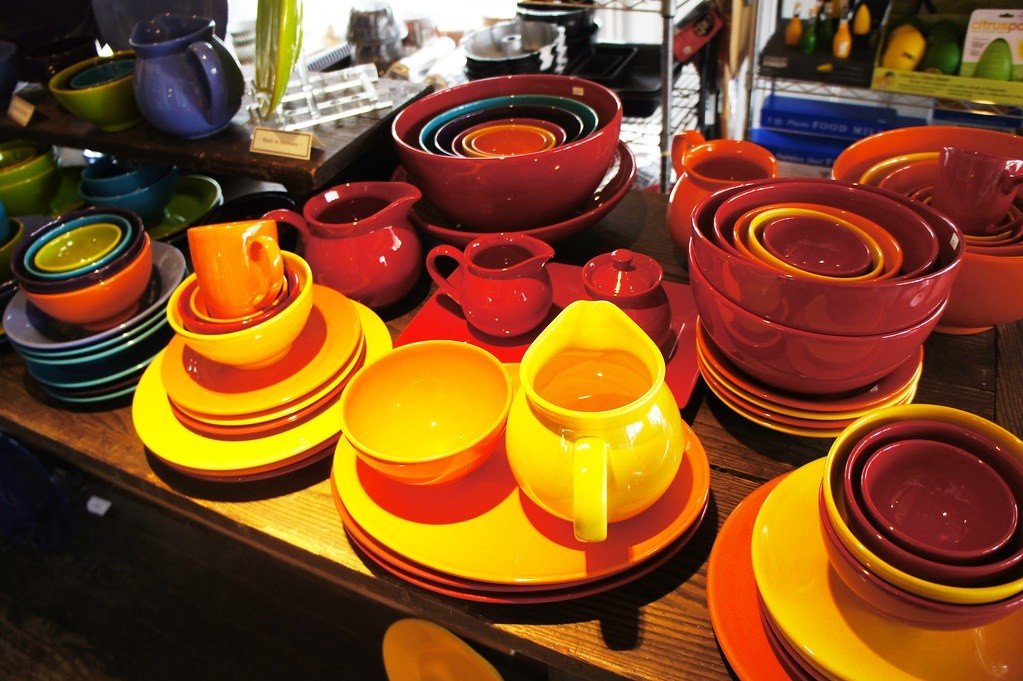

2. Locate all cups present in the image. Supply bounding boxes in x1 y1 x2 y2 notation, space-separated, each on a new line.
187 220 284 319
932 147 1023 236
0 201 11 245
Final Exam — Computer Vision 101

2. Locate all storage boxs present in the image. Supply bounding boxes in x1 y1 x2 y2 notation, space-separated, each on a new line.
740 0 1023 184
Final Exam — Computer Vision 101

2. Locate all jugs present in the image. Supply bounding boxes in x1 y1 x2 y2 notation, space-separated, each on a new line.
259 182 555 338
129 13 244 139
505 300 684 543
666 129 780 265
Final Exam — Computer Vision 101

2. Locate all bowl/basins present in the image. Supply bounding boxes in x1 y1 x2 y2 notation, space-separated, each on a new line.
188 192 299 253
10 206 153 331
166 250 313 369
0 33 136 132
338 340 511 485
688 126 1023 395
390 74 637 244
818 403 1023 624
0 138 56 216
79 149 181 228
0 217 25 279
346 5 409 74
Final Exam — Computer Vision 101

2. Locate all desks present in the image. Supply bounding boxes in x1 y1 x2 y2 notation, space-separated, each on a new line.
0 186 1023 681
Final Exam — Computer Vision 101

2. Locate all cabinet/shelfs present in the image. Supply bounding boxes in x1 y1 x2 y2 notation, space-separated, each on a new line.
588 0 724 195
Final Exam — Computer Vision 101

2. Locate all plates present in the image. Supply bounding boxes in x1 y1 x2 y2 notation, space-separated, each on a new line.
2 242 190 403
131 284 393 483
147 175 224 244
706 456 1023 681
392 262 923 437
331 363 710 603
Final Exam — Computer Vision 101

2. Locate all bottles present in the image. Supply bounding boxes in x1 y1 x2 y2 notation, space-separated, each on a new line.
784 2 871 60
580 249 672 348
